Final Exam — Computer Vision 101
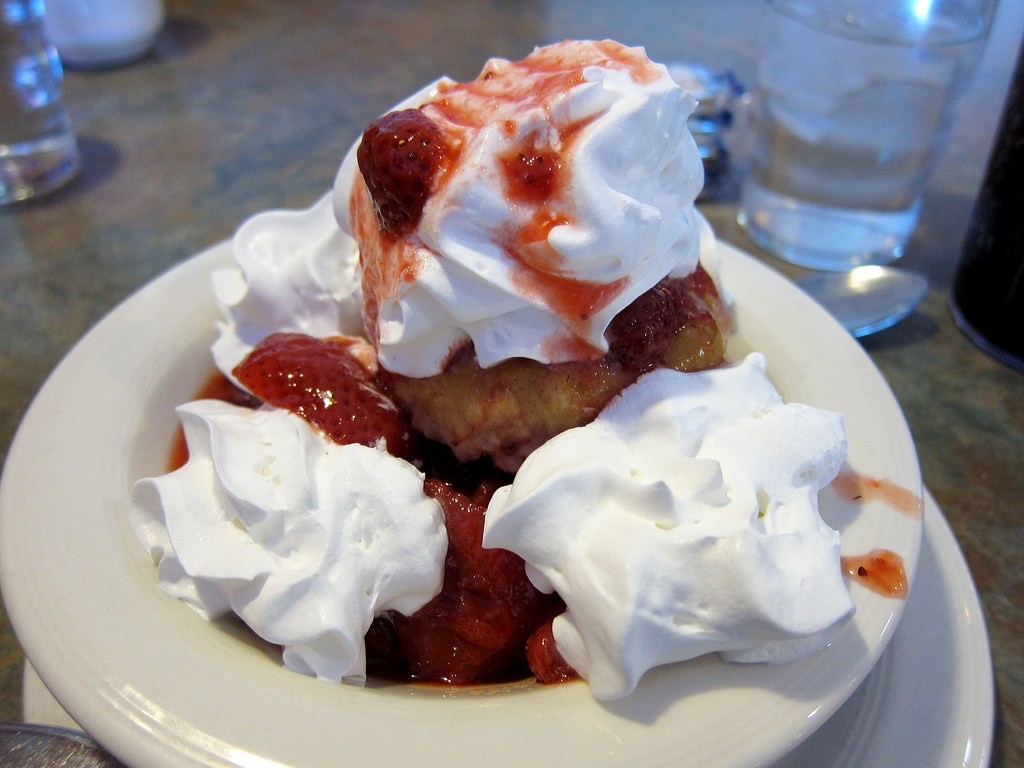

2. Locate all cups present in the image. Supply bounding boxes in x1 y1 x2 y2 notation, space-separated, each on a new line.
531 1 1024 372
0 1 84 206
45 1 166 71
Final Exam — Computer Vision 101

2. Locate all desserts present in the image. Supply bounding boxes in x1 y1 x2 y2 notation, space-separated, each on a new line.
121 31 856 700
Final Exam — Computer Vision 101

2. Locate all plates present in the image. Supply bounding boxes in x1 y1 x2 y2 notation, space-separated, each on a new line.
756 482 996 768
0 216 917 768
